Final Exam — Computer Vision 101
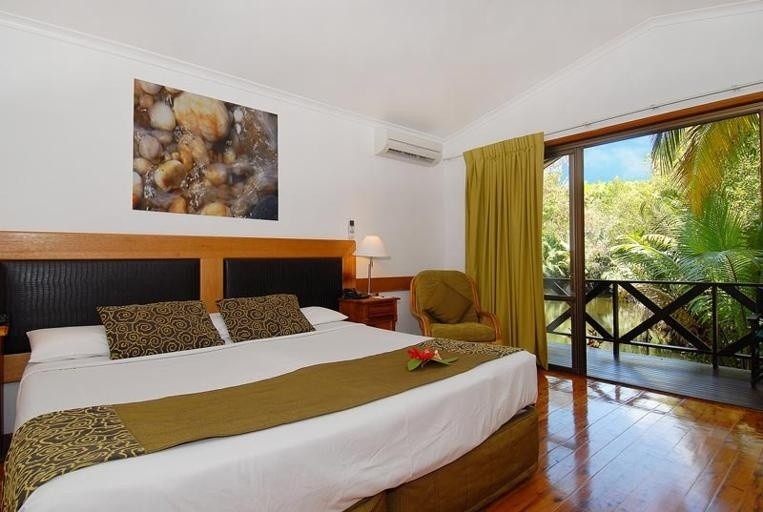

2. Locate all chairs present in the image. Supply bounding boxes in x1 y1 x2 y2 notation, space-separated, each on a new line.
410 270 505 345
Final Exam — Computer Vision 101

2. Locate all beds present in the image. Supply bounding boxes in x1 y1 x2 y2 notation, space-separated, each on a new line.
0 231 539 512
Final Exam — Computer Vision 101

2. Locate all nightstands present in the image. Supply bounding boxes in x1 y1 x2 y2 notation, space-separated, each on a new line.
340 296 400 331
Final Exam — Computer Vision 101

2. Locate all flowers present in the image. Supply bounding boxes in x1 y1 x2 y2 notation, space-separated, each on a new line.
408 344 459 372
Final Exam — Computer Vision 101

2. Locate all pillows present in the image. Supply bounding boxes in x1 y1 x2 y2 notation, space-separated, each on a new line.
215 294 316 343
210 313 230 341
422 281 473 324
98 301 226 360
26 325 110 363
299 306 349 327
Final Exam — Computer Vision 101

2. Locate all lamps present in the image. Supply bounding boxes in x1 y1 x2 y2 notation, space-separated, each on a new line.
352 236 391 296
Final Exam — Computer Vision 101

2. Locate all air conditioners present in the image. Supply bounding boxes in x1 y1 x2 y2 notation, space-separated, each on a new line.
375 126 443 167
